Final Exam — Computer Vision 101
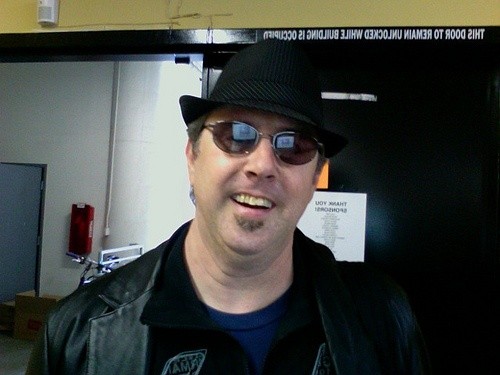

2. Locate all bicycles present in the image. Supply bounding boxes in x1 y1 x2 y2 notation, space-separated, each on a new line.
63 251 120 288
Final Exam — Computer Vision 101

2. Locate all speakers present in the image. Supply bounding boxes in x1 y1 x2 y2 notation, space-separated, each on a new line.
37 0 59 25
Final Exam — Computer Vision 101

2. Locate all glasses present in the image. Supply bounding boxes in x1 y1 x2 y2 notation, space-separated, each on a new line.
201 120 325 165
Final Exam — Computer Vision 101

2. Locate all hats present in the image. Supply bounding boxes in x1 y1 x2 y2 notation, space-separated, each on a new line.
180 39 348 159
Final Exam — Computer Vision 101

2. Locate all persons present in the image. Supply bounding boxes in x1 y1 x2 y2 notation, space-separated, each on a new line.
27 39 424 375
